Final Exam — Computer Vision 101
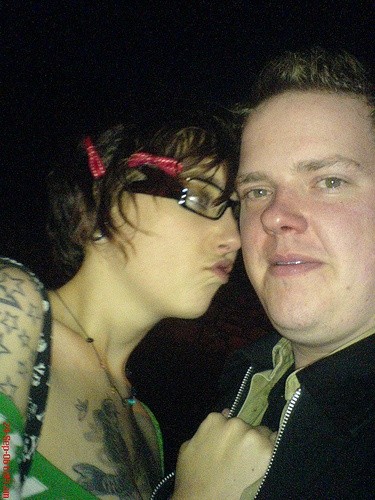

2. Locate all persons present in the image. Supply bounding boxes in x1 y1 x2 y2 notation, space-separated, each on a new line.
161 62 375 499
0 117 279 498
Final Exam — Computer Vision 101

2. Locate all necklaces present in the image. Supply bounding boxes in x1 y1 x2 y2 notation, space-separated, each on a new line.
52 288 138 408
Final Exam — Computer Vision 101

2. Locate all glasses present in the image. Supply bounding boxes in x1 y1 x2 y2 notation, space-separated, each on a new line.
126 171 241 220
